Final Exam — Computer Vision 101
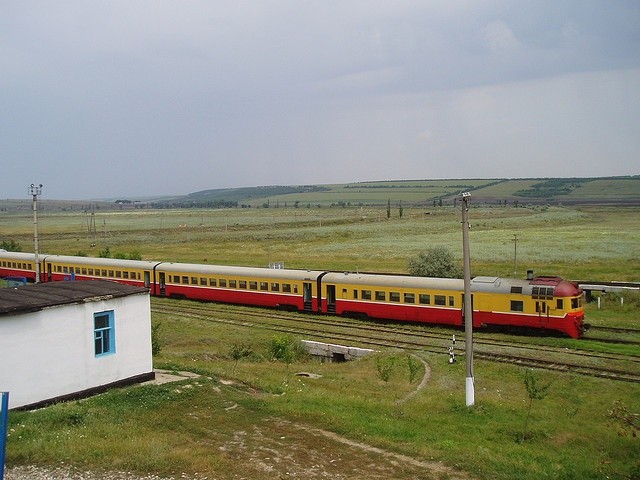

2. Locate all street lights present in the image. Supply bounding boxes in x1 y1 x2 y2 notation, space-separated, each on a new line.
28 184 43 283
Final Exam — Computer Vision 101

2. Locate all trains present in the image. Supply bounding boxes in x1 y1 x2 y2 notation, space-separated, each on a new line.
0 249 591 339
